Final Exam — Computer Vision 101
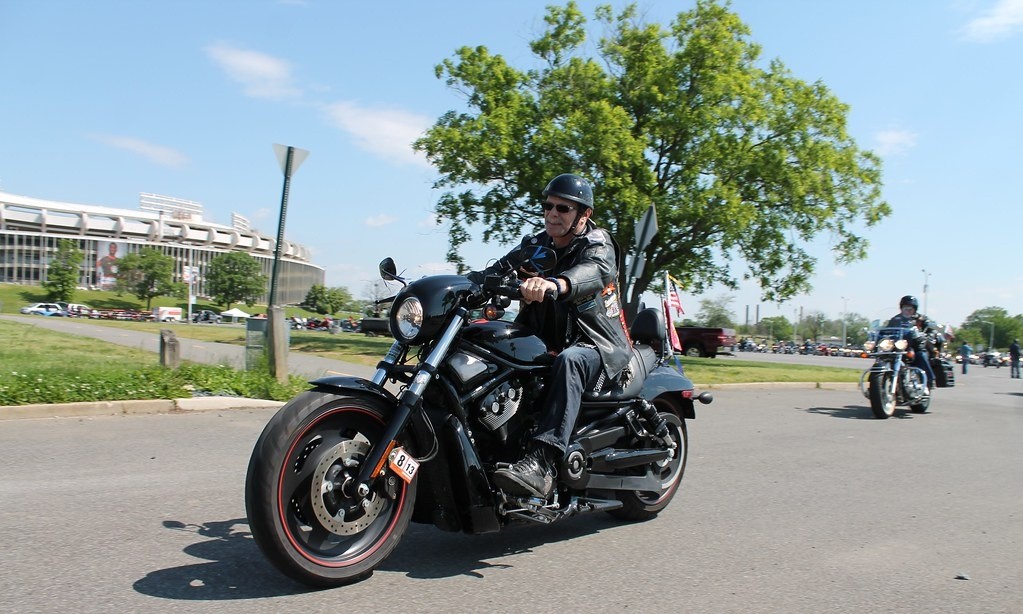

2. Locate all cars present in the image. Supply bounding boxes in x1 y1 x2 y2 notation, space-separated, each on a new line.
20 302 63 317
58 301 91 318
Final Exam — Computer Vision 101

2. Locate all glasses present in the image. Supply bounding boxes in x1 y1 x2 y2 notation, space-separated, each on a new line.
903 307 912 311
542 202 579 213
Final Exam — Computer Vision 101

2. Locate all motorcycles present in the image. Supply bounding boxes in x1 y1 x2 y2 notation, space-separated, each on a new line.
858 319 956 419
737 336 1023 369
48 305 365 334
244 243 715 590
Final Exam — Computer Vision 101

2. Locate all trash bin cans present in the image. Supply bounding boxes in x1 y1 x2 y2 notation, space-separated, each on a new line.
246 318 291 373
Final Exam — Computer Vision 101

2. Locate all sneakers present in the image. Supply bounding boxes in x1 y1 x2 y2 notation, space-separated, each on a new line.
493 442 558 499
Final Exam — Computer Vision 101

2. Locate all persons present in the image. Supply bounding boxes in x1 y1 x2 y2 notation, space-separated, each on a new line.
1010 339 1022 377
739 336 868 358
887 295 938 389
960 341 972 374
464 174 634 498
97 243 118 277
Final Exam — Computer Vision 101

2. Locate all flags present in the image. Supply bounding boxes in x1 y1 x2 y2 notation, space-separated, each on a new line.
663 279 684 351
945 324 955 342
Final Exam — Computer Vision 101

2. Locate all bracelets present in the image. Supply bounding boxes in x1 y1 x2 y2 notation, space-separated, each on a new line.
546 277 561 294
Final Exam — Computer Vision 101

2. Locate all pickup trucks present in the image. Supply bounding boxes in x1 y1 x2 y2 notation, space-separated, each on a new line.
623 301 736 357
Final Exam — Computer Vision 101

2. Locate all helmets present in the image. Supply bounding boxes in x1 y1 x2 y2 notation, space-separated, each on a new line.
900 295 918 309
541 173 594 209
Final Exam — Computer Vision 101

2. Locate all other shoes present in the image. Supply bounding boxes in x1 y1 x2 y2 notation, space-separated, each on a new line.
929 379 936 390
1017 375 1020 378
